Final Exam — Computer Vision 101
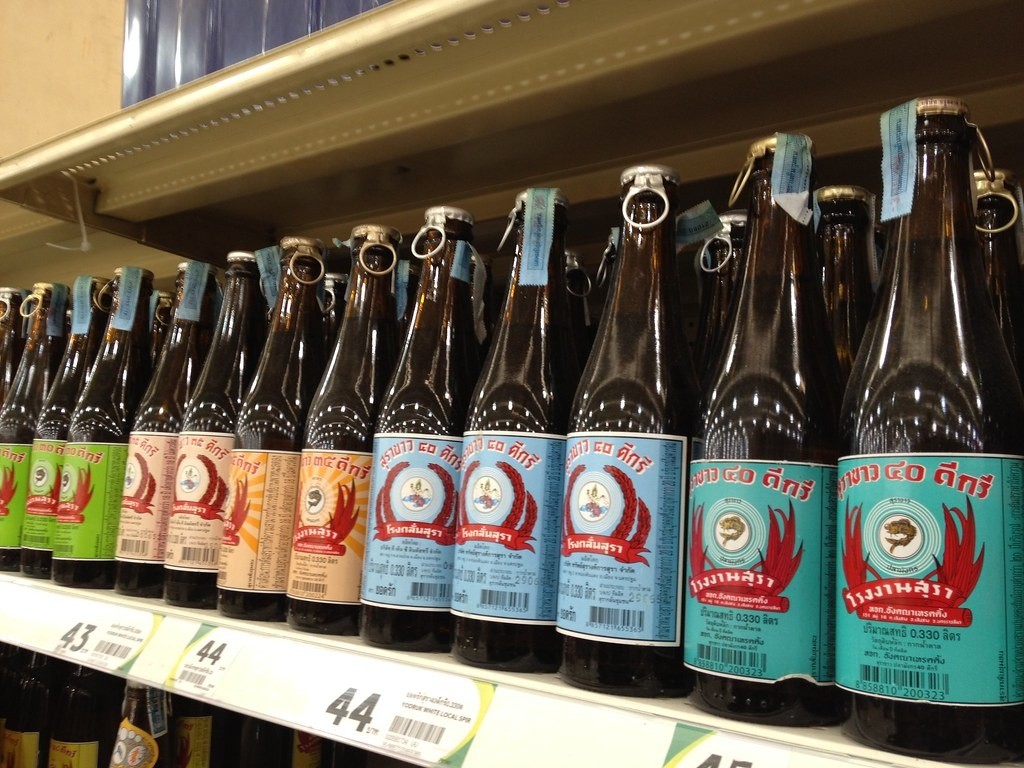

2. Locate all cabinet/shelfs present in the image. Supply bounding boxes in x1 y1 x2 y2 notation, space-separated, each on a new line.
0 0 1024 768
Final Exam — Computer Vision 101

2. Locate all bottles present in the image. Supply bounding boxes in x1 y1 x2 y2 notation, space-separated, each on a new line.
0 96 1024 768
